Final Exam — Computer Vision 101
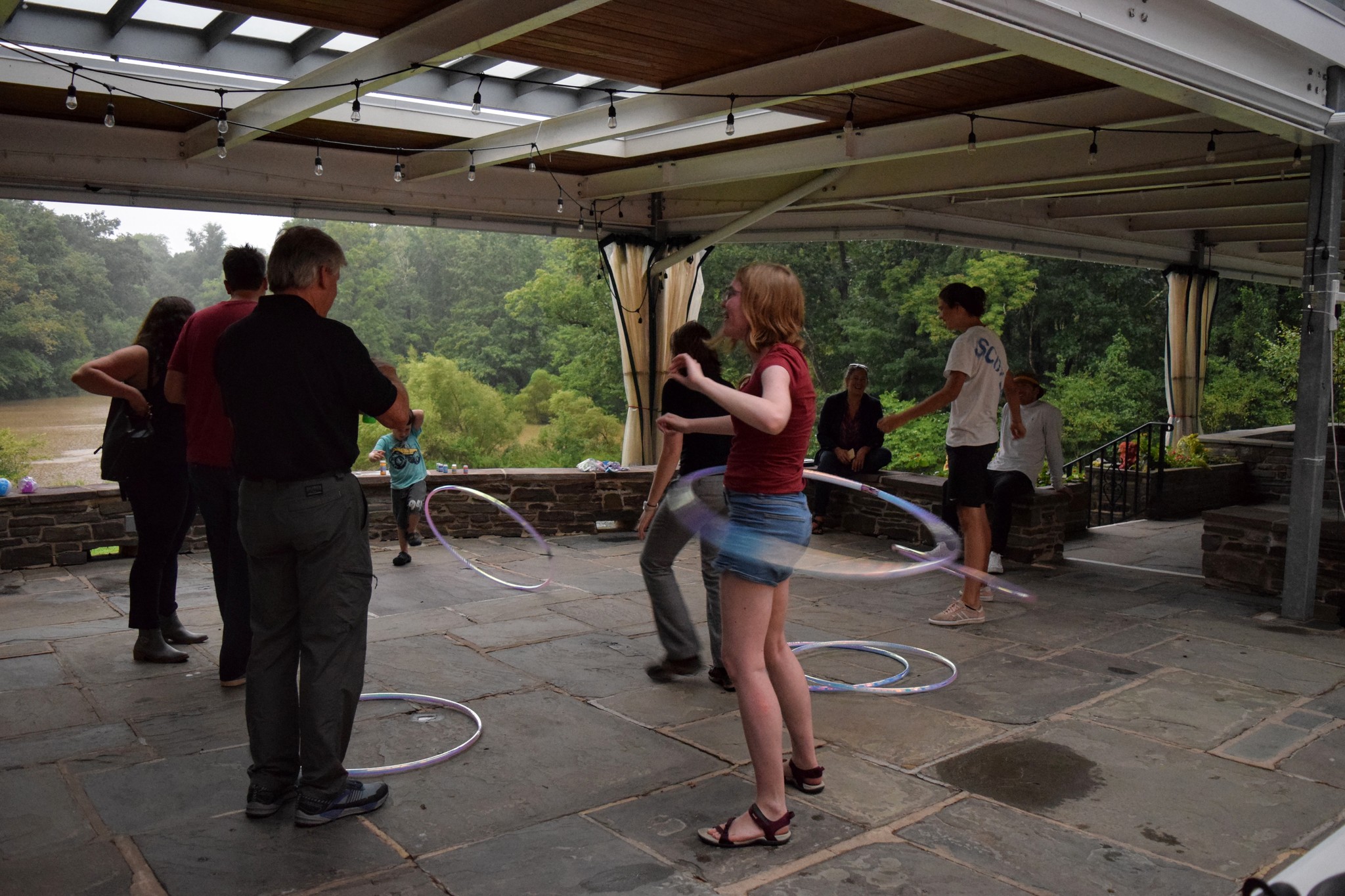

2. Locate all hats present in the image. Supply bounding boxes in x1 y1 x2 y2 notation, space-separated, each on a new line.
1013 372 1046 399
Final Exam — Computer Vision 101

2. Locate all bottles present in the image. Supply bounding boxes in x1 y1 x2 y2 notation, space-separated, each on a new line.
443 465 448 473
463 465 468 474
436 463 441 471
452 464 457 474
439 464 443 472
380 460 387 475
362 412 376 423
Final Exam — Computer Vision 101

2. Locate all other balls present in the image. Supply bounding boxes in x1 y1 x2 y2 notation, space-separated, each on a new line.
19 476 38 493
0 478 12 496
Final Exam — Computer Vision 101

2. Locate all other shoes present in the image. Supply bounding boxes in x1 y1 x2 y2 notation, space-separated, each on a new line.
988 550 1004 574
393 552 412 566
406 531 423 547
645 655 702 679
924 541 964 559
709 667 735 691
220 671 247 686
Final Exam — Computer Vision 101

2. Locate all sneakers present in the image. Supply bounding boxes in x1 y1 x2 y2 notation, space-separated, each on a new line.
293 778 388 825
928 595 985 625
247 783 292 816
959 585 993 601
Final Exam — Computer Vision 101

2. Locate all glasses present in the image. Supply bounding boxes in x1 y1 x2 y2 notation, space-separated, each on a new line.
725 287 741 299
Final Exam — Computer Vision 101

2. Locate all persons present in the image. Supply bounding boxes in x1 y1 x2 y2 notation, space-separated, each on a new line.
656 261 826 849
368 408 426 568
923 371 1074 575
811 363 893 535
165 246 271 689
637 321 739 693
876 282 1026 628
70 296 211 665
212 227 415 829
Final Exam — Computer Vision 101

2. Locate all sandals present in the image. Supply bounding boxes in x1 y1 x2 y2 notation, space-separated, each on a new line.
781 758 825 794
812 519 824 535
697 802 795 847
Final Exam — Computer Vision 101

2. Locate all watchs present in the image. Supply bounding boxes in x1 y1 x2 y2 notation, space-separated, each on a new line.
643 500 659 512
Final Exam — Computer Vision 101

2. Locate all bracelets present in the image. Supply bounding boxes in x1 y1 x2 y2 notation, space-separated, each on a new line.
647 503 657 507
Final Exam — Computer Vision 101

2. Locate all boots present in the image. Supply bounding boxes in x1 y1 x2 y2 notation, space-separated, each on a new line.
163 609 209 643
133 628 189 664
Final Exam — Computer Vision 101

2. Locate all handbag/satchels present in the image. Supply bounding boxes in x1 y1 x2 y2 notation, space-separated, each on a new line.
93 380 154 482
